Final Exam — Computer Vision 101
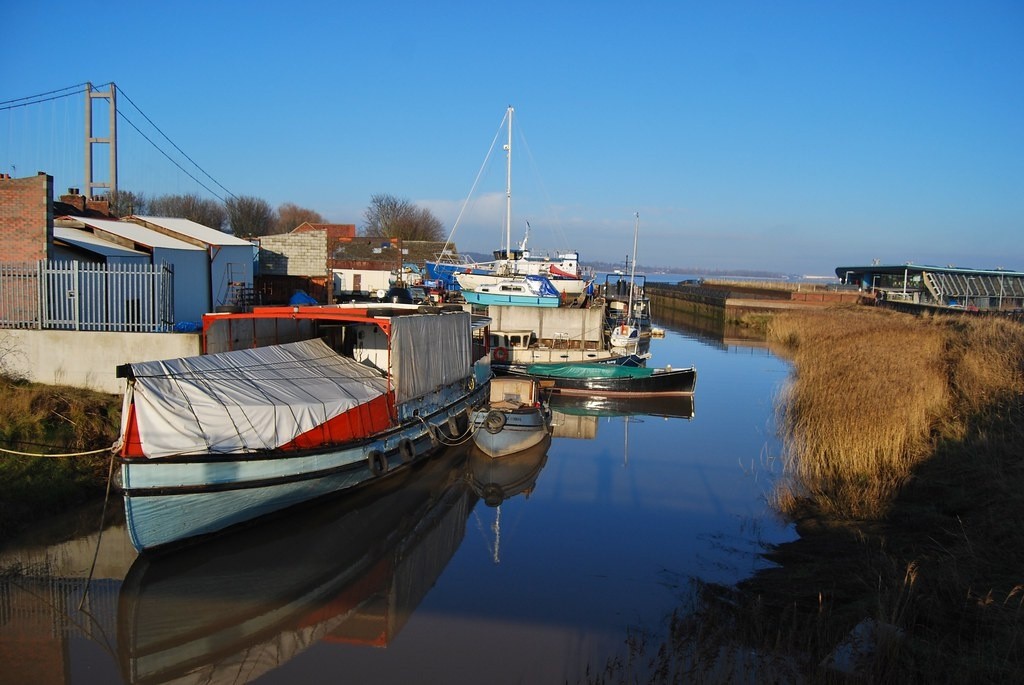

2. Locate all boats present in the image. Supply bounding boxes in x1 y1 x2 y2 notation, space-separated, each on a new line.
493 363 697 397
473 329 651 367
116 301 494 554
465 432 553 507
603 272 651 343
467 375 555 459
462 280 560 308
539 394 694 420
650 328 666 335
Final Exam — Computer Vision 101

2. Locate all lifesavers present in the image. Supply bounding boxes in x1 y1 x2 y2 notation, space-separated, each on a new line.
399 438 416 462
428 424 441 448
494 347 507 361
368 450 388 476
448 416 460 438
484 410 506 434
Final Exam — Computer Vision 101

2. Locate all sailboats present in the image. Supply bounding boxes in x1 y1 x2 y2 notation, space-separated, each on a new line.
423 104 587 303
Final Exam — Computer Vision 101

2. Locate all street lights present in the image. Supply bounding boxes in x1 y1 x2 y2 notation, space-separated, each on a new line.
872 276 880 296
965 278 975 309
846 272 854 284
